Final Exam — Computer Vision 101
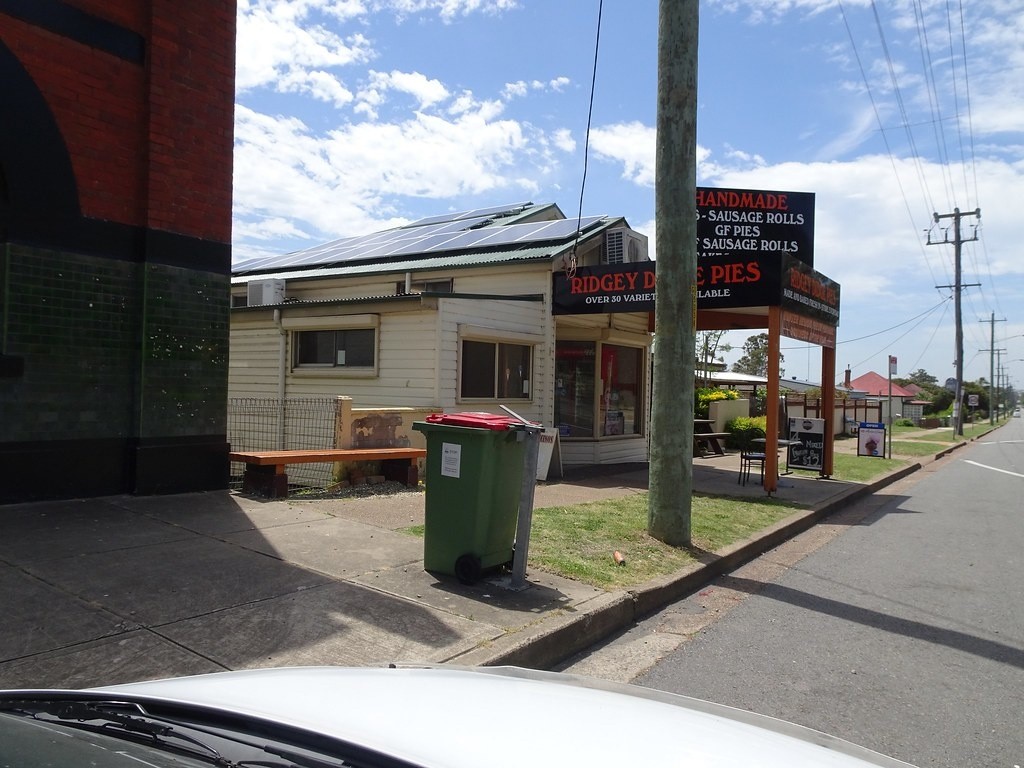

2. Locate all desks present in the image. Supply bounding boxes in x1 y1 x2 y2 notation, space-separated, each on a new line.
692 419 716 424
751 437 802 475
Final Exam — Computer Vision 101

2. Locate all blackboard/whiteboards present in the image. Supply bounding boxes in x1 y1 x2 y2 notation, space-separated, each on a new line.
786 417 825 471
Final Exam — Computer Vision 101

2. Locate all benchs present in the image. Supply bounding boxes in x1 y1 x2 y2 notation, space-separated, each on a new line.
230 447 428 497
694 432 732 457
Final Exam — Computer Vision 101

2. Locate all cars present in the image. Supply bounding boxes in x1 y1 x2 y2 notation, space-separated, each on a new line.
1013 409 1021 417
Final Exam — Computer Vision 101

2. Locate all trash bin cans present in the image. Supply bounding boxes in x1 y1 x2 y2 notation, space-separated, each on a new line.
408 411 545 588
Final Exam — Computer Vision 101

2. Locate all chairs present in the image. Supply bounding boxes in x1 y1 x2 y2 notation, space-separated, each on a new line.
735 427 780 486
